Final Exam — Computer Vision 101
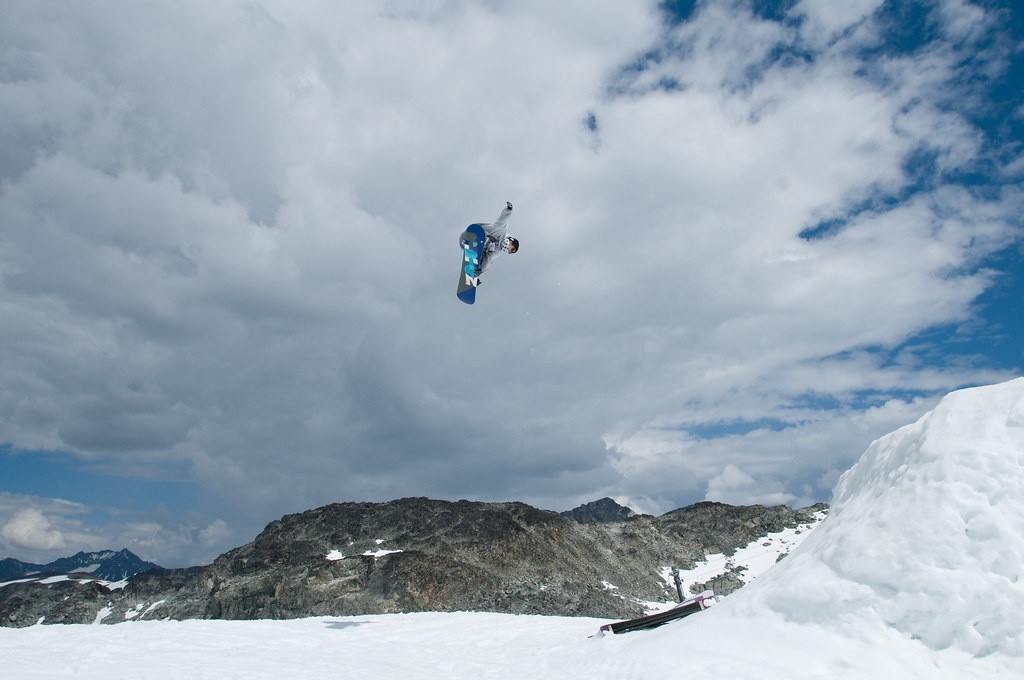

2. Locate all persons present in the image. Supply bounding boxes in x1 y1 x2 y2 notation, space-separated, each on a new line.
471 202 519 286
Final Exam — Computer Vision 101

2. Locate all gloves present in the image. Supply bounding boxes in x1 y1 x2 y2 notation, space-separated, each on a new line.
507 202 512 210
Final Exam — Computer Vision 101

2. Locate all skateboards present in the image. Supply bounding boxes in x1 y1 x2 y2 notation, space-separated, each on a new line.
455 223 487 306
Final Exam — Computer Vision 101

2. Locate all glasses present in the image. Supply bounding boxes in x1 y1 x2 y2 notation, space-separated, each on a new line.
511 241 516 252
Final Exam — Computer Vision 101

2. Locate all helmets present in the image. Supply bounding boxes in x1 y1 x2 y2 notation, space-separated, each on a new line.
508 237 519 253
